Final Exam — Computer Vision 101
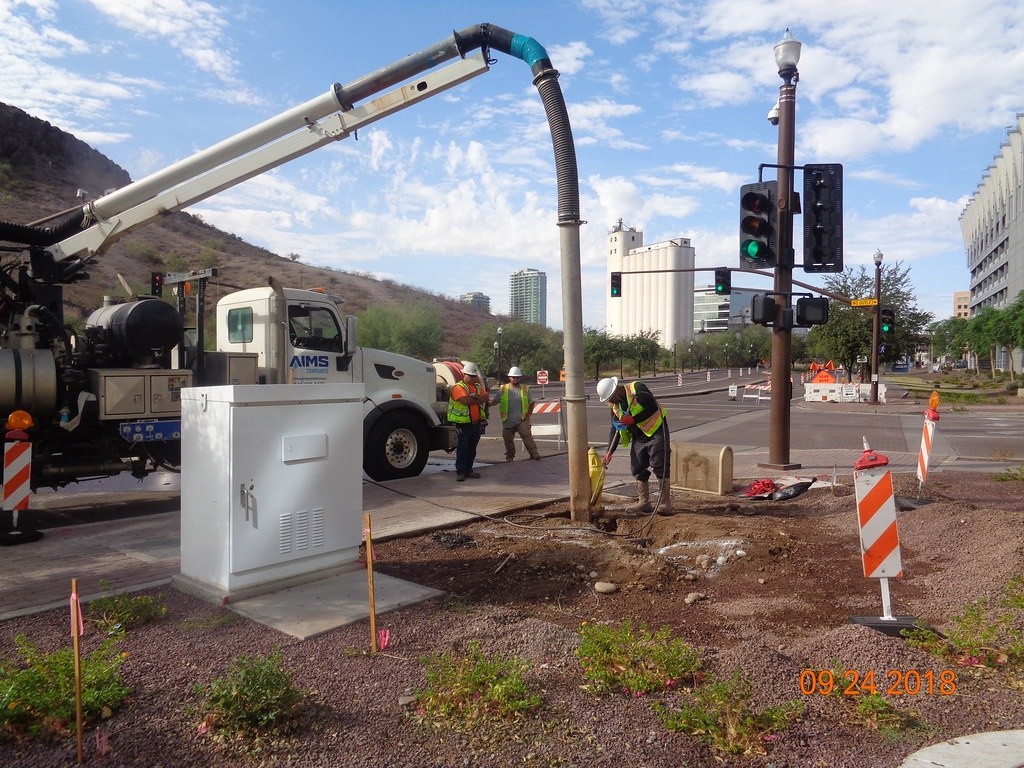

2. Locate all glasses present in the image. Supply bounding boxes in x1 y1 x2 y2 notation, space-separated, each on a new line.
511 376 519 379
465 374 477 379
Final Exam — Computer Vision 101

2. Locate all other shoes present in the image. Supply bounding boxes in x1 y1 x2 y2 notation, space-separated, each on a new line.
506 456 513 462
465 471 481 477
530 452 540 460
456 471 465 480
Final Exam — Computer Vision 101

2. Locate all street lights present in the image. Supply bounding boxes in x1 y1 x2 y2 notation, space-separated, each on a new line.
866 247 884 405
757 28 803 470
723 342 729 369
670 340 678 374
688 340 694 372
748 343 753 367
705 341 710 370
493 326 503 388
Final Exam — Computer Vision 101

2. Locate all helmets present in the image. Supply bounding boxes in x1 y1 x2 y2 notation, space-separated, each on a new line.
507 366 523 377
460 362 478 375
597 376 618 402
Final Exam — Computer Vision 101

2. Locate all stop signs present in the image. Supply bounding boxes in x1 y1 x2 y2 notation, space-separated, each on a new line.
536 370 548 385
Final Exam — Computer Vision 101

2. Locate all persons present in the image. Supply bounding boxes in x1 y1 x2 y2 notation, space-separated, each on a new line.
488 367 540 462
596 376 673 515
446 362 491 481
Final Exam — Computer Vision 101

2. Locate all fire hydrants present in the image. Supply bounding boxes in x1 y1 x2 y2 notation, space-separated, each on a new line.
587 446 607 513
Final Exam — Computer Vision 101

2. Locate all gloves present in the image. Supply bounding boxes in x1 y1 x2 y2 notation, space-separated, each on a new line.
602 453 612 469
620 415 634 427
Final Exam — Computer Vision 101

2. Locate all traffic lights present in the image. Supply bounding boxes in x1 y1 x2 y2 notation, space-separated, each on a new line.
880 307 894 336
738 179 780 273
610 272 622 298
750 294 775 324
713 266 731 296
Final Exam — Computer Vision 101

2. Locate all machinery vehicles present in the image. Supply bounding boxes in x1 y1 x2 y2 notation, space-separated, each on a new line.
0 21 595 523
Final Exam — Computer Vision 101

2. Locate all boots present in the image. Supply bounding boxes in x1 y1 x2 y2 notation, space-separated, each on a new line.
625 480 651 513
657 478 672 515
